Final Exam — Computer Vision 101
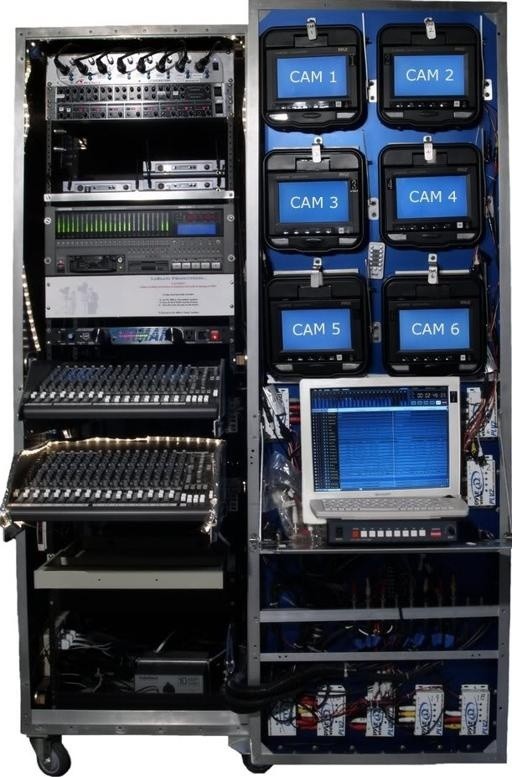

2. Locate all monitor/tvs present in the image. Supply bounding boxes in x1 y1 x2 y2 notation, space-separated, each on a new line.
299 376 461 525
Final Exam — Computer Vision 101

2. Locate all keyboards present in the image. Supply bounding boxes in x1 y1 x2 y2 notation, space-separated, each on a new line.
310 497 468 520
